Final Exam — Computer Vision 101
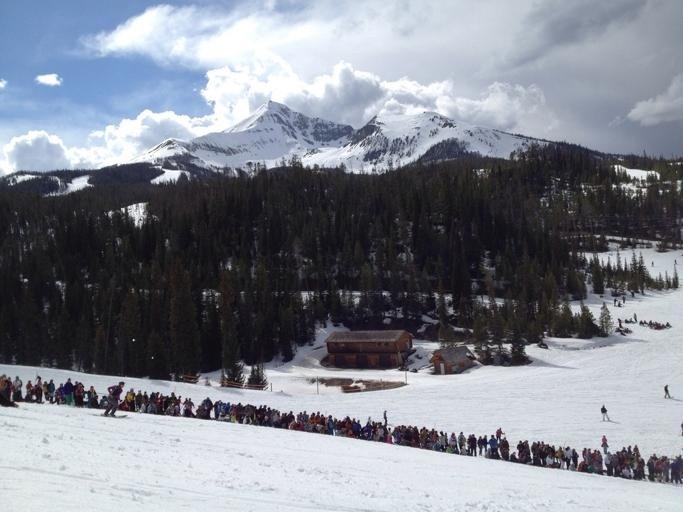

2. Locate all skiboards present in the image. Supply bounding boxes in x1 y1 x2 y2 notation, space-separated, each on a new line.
94 413 128 418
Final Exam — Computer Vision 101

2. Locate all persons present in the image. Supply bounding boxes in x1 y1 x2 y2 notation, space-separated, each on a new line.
614 288 671 337
